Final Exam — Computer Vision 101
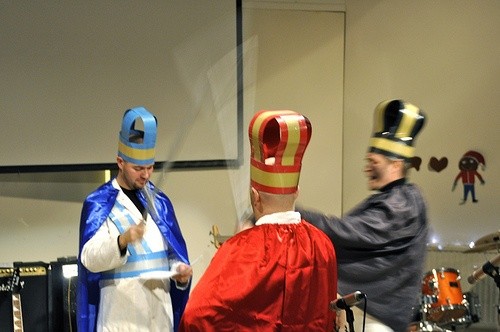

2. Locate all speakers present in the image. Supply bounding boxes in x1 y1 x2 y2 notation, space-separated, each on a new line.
0 260 79 332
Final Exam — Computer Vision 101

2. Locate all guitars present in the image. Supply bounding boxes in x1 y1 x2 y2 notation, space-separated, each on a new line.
6 266 24 332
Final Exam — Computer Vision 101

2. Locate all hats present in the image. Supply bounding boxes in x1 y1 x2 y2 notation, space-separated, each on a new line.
117 106 157 166
368 99 426 165
250 110 312 194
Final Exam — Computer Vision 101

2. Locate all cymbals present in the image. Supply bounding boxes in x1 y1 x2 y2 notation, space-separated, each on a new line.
475 231 500 245
462 242 500 253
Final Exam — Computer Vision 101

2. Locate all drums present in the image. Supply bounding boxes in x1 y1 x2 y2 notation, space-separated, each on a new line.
422 268 469 327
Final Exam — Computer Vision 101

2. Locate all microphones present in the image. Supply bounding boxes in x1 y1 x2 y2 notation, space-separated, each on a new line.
468 254 500 285
330 291 366 312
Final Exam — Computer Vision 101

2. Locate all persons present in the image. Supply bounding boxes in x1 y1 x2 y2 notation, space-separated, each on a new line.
236 98 428 332
78 106 193 332
178 110 337 332
